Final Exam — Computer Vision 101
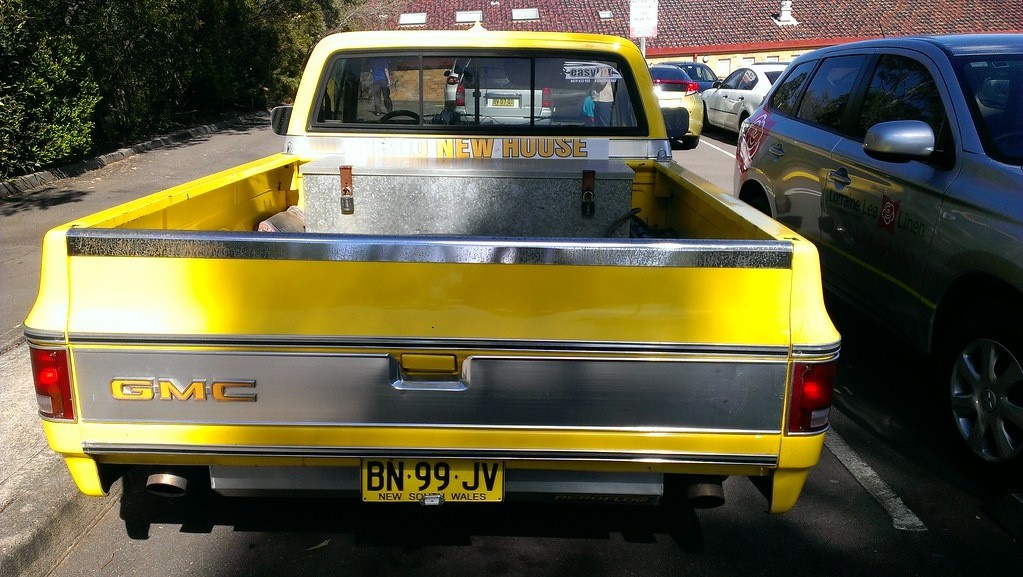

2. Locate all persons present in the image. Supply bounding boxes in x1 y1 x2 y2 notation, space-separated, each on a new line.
372 56 393 115
590 64 614 128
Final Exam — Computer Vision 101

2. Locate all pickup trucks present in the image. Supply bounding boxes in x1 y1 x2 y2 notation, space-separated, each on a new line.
22 20 843 513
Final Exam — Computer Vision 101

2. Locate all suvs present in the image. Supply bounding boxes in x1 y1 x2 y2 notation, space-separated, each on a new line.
731 32 1023 495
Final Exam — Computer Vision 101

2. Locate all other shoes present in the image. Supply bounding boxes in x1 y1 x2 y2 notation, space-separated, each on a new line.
376 113 381 116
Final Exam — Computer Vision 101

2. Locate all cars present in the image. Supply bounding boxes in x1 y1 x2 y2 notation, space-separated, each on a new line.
440 56 470 112
656 59 720 94
701 61 789 136
645 62 704 148
452 56 555 125
